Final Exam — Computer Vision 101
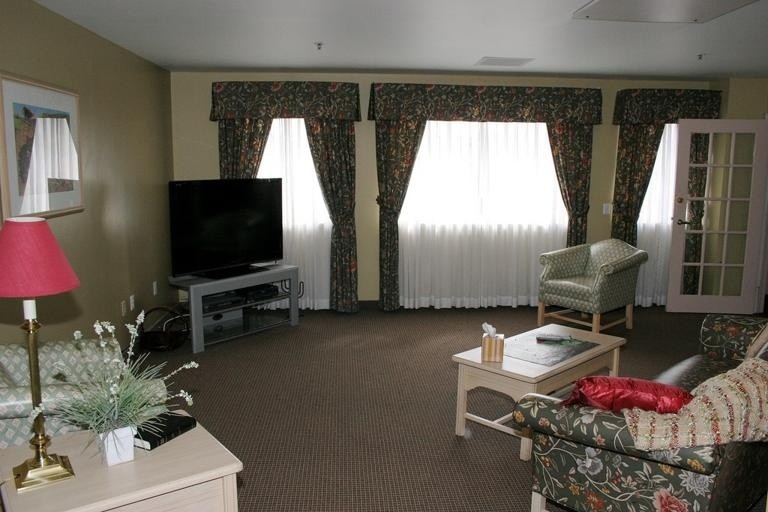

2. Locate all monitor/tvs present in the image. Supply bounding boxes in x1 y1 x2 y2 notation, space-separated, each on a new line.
168 178 283 280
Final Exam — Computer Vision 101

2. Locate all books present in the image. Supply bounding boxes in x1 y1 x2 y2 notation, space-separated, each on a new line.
133 411 197 452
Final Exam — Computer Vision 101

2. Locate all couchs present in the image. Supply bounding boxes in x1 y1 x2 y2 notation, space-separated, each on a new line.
512 319 768 512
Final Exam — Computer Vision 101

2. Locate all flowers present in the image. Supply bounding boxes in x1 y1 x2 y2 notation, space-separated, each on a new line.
30 308 200 463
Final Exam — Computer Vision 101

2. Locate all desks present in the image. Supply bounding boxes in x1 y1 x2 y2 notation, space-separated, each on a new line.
0 404 244 512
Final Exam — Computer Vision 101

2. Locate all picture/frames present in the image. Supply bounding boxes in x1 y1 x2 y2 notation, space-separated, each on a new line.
0 71 86 225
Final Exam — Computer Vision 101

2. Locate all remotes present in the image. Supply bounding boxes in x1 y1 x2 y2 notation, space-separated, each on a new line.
537 334 563 343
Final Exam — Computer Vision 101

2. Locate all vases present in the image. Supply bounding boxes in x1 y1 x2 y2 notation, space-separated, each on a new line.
90 423 135 468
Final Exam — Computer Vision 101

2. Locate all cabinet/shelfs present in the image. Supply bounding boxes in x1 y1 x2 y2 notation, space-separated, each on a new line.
168 261 299 355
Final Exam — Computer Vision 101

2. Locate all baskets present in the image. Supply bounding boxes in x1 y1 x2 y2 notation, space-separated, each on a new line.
140 307 190 352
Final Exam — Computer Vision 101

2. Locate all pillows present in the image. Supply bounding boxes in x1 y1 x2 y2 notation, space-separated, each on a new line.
558 374 695 414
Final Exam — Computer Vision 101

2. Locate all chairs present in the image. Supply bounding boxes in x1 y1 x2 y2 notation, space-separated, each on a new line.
537 236 649 334
0 337 169 451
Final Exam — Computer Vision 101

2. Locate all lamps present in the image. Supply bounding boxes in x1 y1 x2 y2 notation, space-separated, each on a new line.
0 215 81 494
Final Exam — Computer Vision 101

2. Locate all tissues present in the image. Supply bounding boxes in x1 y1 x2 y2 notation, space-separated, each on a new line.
480 321 505 363
